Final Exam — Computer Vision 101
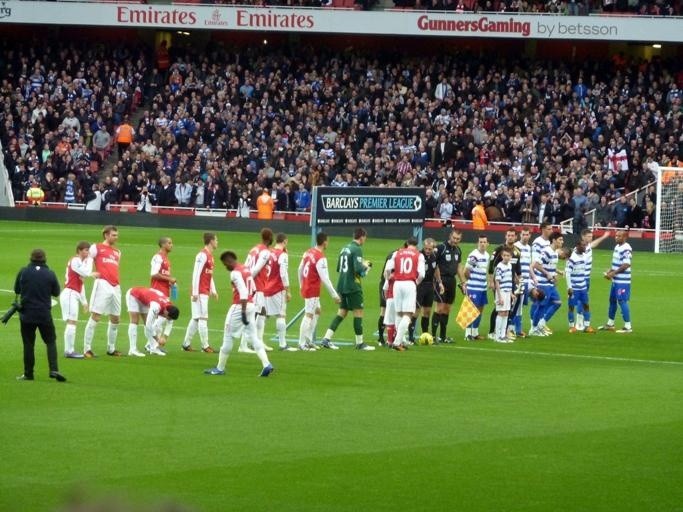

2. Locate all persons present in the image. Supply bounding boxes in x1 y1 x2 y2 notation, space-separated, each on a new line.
487 229 523 340
494 249 515 343
203 251 274 377
239 227 274 354
126 286 178 357
531 223 553 335
14 249 67 382
565 240 594 333
320 228 375 350
378 240 408 346
409 238 444 346
597 230 633 333
433 230 466 346
60 241 90 359
571 228 611 331
464 236 490 341
182 232 218 353
223 0 683 19
145 236 177 356
298 230 341 351
514 228 539 338
265 234 297 352
383 270 394 348
0 40 682 227
528 281 545 302
84 225 122 358
528 232 563 336
384 236 425 351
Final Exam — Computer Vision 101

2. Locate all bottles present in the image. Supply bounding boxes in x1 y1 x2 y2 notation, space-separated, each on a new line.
171 281 177 302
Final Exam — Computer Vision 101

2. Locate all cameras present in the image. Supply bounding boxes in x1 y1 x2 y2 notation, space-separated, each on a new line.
1 302 25 325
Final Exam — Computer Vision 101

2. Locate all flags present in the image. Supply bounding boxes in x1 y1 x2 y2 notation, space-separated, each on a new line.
608 148 628 173
455 283 481 330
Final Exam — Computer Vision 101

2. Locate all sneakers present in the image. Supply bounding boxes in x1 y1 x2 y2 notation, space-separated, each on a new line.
615 328 632 333
83 351 96 357
128 348 145 357
150 347 165 355
310 343 320 349
476 335 484 339
494 338 509 343
279 345 297 351
259 363 272 376
528 329 545 336
321 339 339 349
391 345 408 352
181 344 195 351
16 375 34 381
583 325 597 332
355 343 375 351
204 367 224 376
599 324 616 330
236 347 253 353
48 371 66 381
440 337 454 343
105 349 122 357
66 352 84 359
464 335 475 340
262 344 273 352
407 340 418 345
298 346 315 351
200 345 217 352
